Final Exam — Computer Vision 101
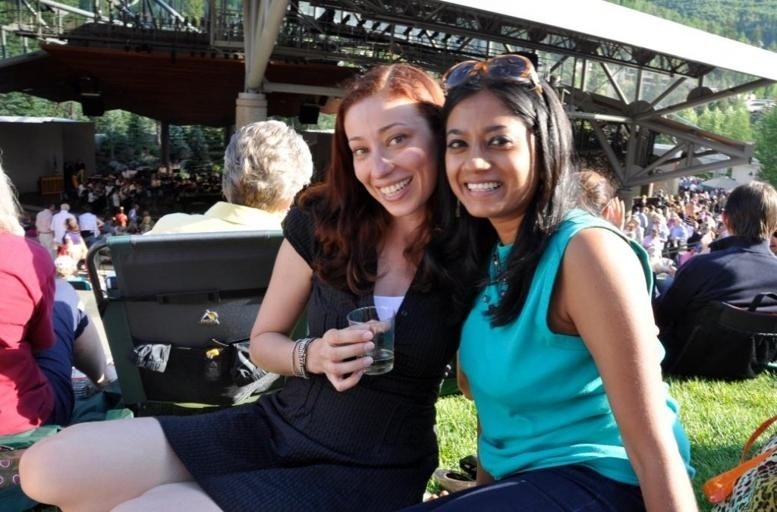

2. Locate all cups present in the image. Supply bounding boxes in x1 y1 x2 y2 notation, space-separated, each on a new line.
345 304 397 376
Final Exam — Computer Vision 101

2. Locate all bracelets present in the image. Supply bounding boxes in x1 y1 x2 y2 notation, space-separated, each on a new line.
290 337 300 377
298 338 315 377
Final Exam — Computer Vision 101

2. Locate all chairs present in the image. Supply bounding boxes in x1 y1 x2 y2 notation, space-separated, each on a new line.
86 231 284 417
663 300 777 382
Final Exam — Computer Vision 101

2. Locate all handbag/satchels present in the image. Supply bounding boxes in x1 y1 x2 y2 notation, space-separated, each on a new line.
702 414 776 512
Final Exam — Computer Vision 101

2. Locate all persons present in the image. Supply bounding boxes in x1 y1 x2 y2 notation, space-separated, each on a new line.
408 54 702 511
623 183 730 298
563 169 626 231
654 181 775 381
34 169 222 281
146 118 316 402
15 61 500 510
1 171 111 447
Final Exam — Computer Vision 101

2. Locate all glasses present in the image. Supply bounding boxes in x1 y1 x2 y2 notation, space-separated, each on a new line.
442 54 539 90
